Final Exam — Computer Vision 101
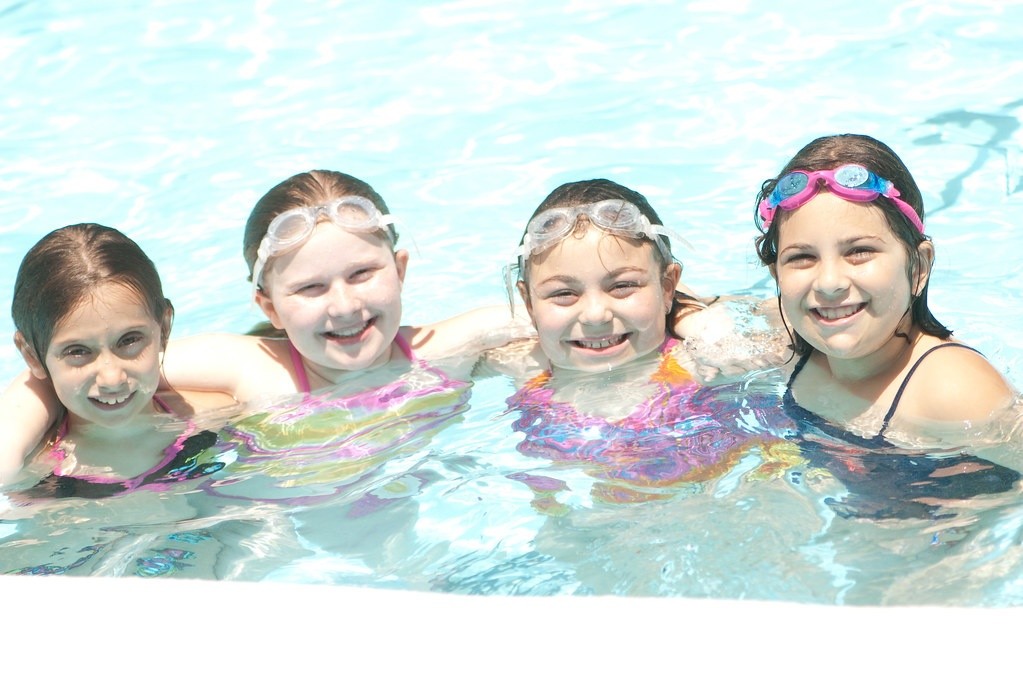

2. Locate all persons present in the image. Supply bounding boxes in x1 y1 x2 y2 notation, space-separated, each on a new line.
1 221 238 582
705 134 1022 608
0 169 750 585
427 178 793 513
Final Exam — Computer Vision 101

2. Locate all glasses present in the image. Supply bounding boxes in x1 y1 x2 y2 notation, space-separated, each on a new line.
516 198 672 278
758 164 924 247
249 195 397 290
